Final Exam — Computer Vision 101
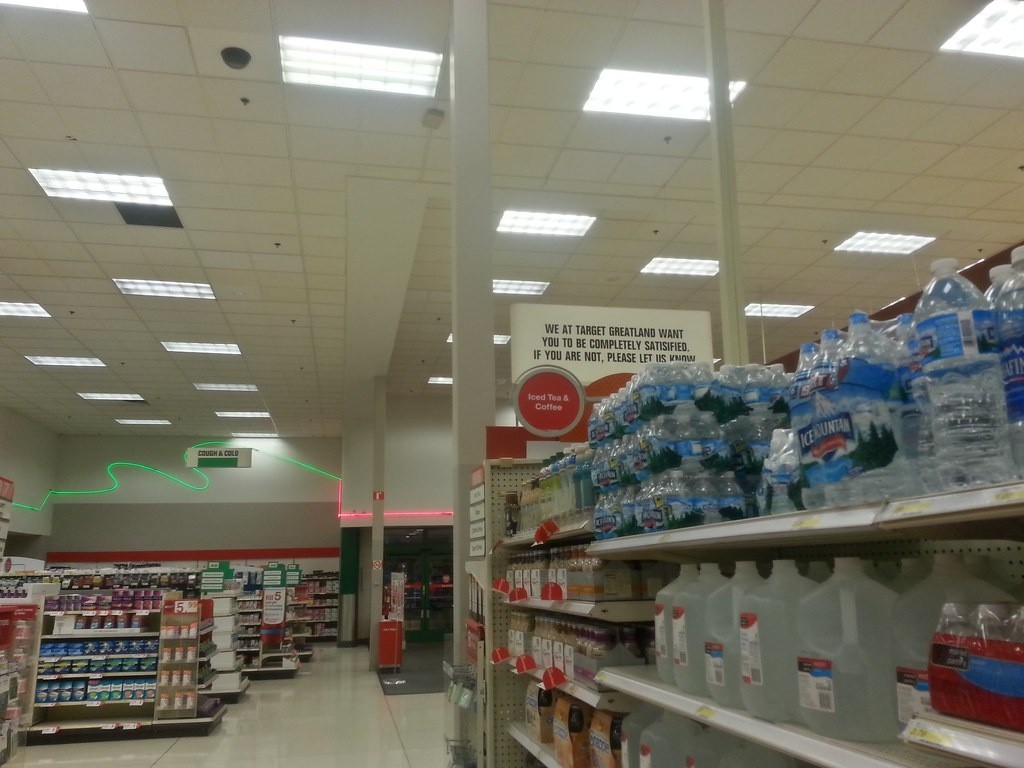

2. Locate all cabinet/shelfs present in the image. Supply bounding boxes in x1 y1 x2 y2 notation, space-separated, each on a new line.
294 585 313 662
237 588 301 681
484 457 1024 768
0 597 227 746
198 592 251 704
302 575 339 642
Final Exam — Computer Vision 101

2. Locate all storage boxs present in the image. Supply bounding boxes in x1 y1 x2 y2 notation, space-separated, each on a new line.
573 625 646 692
525 679 624 768
35 590 244 702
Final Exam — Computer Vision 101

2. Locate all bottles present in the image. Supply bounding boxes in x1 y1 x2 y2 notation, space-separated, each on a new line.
895 549 1024 725
655 559 898 741
621 703 663 768
525 683 622 768
504 442 589 536
508 610 553 670
549 545 606 602
790 255 1024 514
589 372 636 542
638 363 795 536
638 708 791 768
505 549 547 600
555 620 615 693
